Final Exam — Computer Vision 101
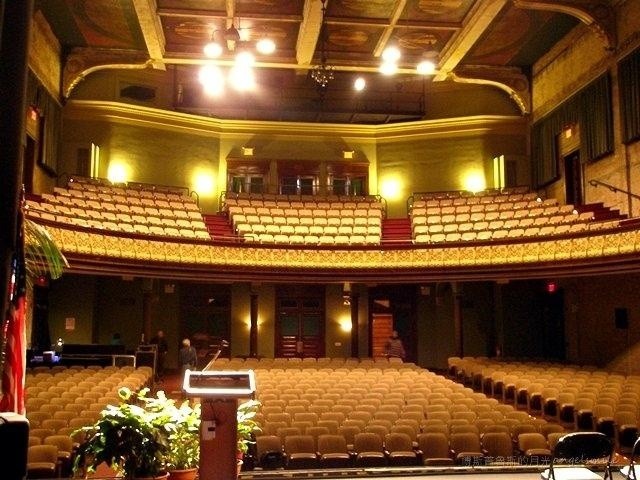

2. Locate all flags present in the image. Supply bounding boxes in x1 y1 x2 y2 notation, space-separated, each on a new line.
0 260 28 419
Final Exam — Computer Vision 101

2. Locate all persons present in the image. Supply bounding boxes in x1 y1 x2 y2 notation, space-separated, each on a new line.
108 332 124 345
176 338 199 375
149 329 168 378
382 328 408 362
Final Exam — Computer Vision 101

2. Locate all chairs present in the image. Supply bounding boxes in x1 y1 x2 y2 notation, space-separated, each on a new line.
22 176 623 244
24 356 640 480
